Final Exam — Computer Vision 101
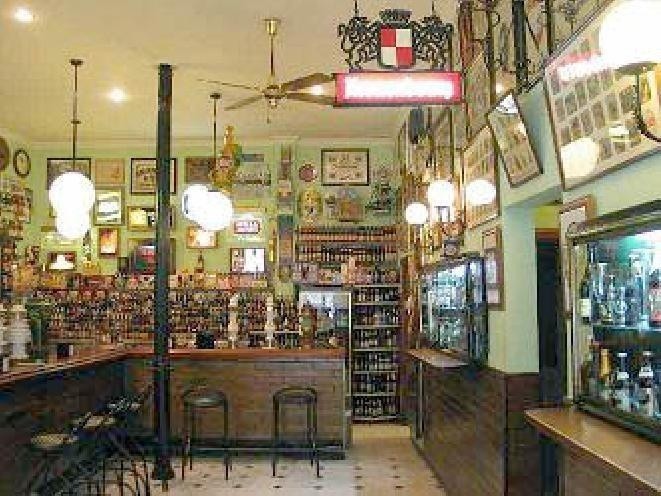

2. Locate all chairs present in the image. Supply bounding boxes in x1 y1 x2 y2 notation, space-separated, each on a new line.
21 381 153 496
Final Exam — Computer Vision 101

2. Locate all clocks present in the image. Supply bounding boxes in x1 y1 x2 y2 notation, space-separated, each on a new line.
15 148 32 177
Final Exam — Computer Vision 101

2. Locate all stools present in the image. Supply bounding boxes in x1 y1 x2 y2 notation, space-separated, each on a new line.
181 386 233 478
269 387 321 479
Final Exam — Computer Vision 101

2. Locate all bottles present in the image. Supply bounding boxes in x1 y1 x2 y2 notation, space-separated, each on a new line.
0 339 10 374
575 252 660 425
293 221 400 418
